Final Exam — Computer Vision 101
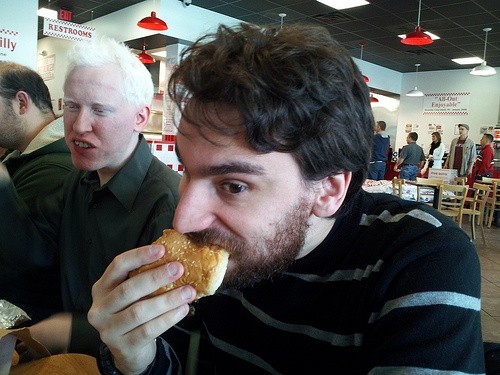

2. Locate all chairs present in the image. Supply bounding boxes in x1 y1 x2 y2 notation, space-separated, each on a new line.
392 176 500 246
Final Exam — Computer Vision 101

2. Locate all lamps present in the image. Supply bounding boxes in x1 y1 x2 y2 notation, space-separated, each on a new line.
406 64 424 96
401 0 433 45
136 43 156 63
470 28 496 76
369 94 379 102
137 0 168 30
360 44 369 82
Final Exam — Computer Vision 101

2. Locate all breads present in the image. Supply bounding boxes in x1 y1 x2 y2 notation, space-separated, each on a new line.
129 228 229 302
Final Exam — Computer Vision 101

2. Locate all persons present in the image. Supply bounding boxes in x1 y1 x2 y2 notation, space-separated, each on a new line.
0 36 495 358
87 22 486 375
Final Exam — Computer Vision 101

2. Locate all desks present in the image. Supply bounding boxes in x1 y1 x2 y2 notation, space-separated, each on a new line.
362 179 455 210
